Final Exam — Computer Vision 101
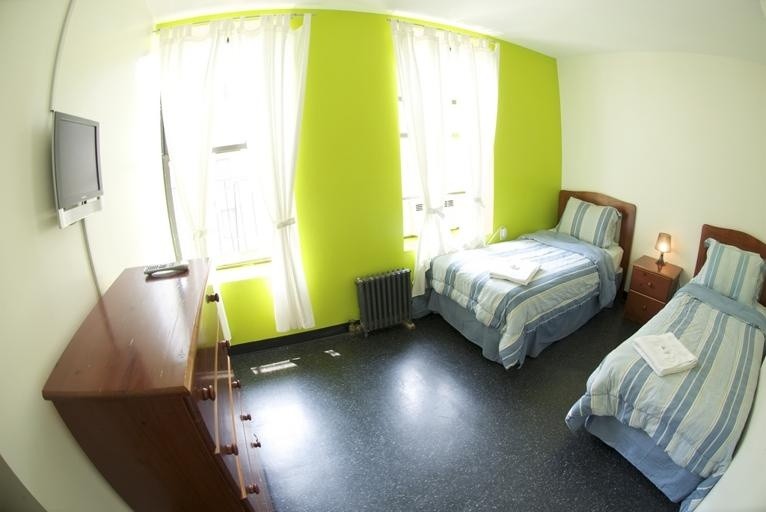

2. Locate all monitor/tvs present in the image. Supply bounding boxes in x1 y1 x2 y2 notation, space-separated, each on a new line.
52 111 103 209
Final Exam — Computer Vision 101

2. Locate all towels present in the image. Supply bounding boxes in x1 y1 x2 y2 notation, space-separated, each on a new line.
634 332 698 377
489 260 541 286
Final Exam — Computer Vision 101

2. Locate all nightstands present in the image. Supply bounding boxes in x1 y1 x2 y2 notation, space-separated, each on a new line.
623 256 682 325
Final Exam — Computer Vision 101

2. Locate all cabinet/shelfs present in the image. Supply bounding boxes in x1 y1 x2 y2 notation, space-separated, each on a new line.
42 258 276 512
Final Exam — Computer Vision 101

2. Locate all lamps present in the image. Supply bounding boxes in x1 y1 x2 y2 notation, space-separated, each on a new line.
654 233 671 267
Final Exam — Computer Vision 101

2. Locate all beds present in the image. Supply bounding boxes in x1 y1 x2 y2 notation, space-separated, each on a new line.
564 224 766 512
425 190 636 372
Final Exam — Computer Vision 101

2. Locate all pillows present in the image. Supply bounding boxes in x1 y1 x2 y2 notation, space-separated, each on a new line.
690 238 766 308
556 196 622 249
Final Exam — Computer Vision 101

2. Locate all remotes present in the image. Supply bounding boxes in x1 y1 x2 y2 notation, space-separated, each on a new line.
144 260 190 274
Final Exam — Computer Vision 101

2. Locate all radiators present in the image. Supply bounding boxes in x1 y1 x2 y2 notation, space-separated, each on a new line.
354 270 413 338
